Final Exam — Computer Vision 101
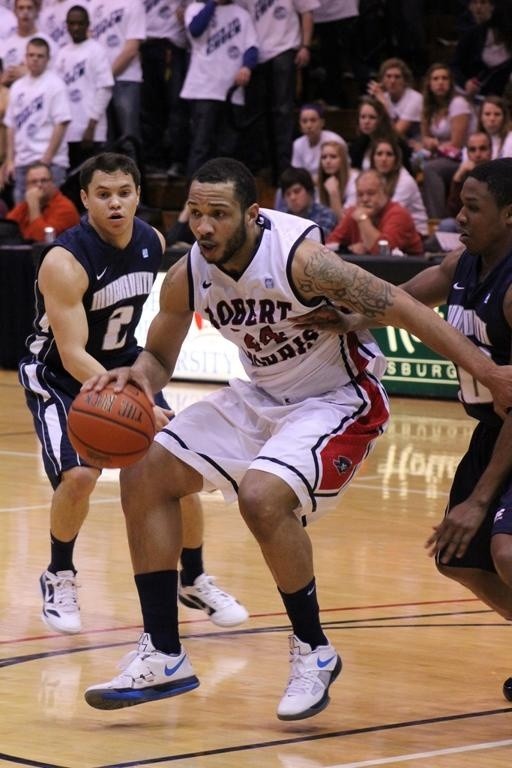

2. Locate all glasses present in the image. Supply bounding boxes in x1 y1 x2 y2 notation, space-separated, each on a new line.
26 178 53 184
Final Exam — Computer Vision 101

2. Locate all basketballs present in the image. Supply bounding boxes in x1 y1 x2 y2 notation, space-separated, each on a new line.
67 382 156 469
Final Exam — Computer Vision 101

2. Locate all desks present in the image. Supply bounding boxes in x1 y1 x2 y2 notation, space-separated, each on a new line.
1 247 469 404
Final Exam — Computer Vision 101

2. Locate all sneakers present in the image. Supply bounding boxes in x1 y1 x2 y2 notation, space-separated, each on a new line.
177 573 250 626
84 633 200 710
38 569 83 636
276 634 341 720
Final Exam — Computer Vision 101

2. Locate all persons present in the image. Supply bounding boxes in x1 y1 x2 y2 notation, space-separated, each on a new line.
19 153 249 633
0 1 512 255
289 157 512 702
80 156 511 720
